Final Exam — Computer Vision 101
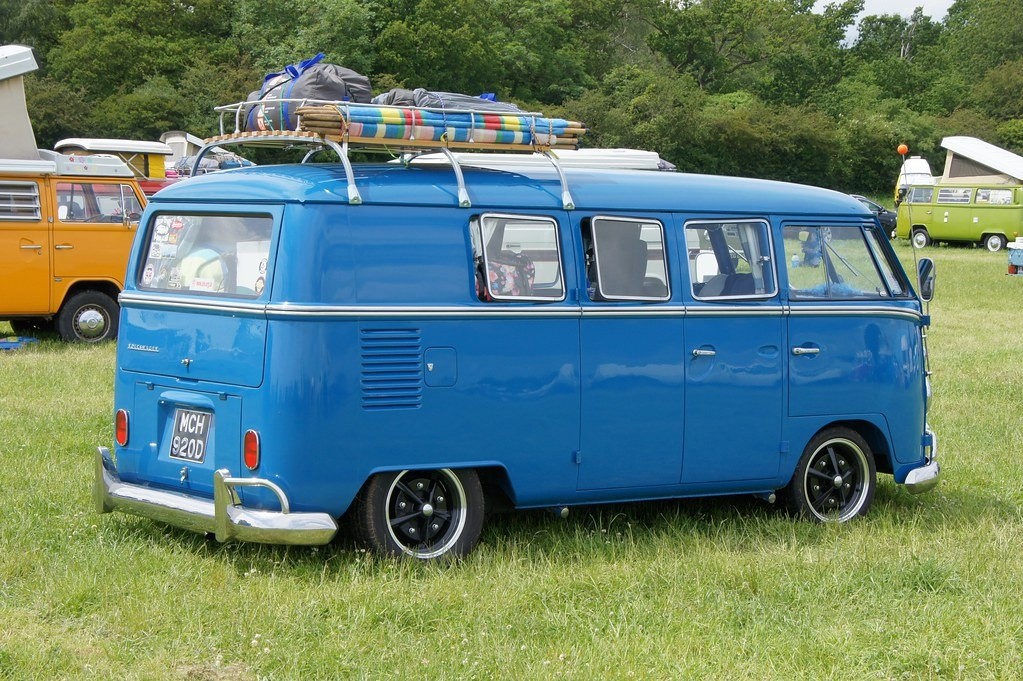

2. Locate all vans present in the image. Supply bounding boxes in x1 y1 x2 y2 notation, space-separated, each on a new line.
894 184 1023 254
93 98 941 572
53 181 183 234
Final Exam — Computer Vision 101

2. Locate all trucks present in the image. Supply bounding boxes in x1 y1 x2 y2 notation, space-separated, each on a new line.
1 43 154 347
894 156 934 210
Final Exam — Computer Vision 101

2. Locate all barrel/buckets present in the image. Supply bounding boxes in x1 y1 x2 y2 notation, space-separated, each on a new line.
1006 242 1023 275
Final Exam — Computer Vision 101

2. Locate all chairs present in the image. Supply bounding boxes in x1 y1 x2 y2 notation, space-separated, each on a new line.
632 234 669 299
58 201 85 219
697 273 757 297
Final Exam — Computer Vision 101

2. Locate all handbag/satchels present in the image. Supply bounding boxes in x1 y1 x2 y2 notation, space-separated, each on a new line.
240 52 371 130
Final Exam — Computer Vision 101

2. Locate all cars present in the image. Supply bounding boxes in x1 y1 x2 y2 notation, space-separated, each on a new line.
165 167 177 175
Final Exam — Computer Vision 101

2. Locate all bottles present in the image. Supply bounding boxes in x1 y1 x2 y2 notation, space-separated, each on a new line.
791 253 799 267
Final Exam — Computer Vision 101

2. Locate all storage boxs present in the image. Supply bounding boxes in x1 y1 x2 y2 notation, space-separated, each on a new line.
1007 242 1023 266
236 232 272 296
